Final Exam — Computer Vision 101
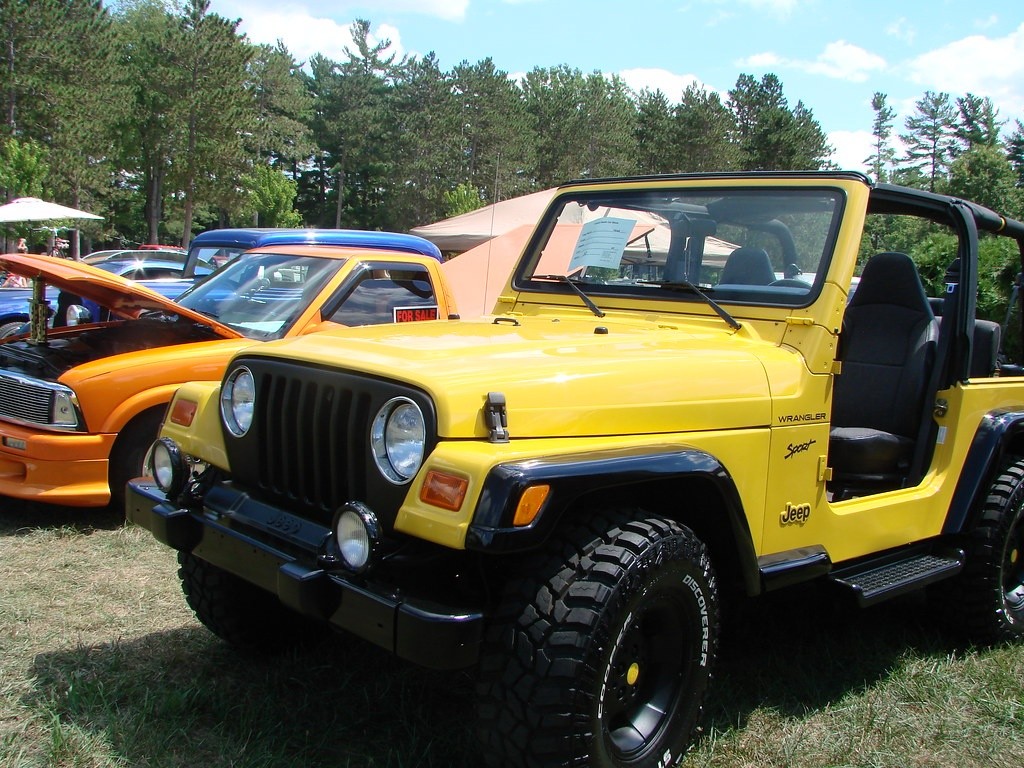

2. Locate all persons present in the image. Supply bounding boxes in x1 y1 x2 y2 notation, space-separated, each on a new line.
2 238 28 288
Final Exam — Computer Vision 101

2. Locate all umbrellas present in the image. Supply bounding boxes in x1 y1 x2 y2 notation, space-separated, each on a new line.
0 197 105 253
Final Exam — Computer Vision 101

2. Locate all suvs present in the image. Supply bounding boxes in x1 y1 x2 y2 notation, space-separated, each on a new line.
125 168 1024 767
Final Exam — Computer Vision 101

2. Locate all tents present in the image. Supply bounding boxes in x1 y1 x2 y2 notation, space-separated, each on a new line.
412 187 742 283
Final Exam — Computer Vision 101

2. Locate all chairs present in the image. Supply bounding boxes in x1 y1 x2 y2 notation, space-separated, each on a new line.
829 252 941 473
719 246 777 285
970 319 1002 378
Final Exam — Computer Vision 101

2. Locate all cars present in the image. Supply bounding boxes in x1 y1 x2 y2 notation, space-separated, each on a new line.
0 227 460 526
